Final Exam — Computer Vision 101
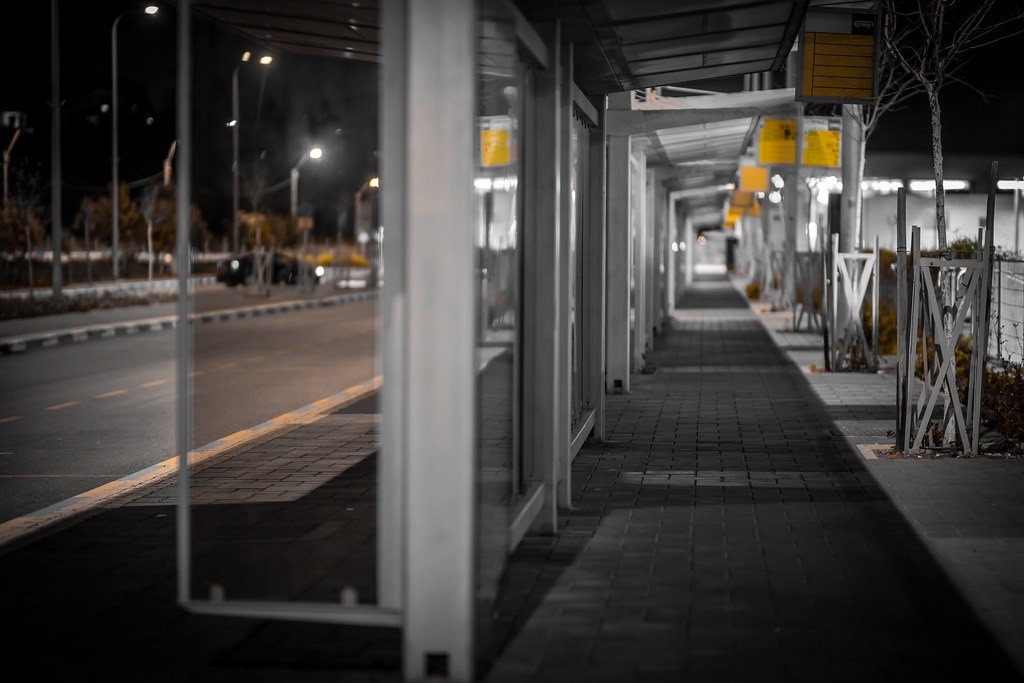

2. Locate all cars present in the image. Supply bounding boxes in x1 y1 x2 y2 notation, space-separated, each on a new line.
215 251 320 287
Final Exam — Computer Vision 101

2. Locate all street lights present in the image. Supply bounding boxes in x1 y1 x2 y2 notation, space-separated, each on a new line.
112 6 158 282
354 178 380 255
232 56 272 254
290 147 322 220
